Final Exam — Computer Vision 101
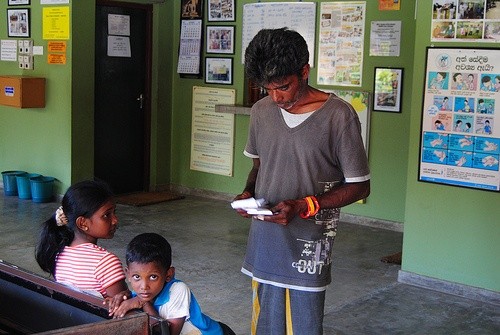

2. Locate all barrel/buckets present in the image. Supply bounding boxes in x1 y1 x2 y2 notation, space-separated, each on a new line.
15 174 41 199
29 176 55 203
1 171 27 196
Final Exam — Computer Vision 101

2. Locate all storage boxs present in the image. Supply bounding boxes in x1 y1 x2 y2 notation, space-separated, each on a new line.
0 76 46 108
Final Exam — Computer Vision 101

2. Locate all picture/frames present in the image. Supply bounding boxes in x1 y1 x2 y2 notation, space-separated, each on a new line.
8 0 31 6
7 8 31 38
372 67 405 113
204 56 234 85
207 0 236 22
206 25 236 55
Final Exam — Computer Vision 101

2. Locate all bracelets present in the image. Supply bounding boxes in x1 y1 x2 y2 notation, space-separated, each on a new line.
300 195 319 219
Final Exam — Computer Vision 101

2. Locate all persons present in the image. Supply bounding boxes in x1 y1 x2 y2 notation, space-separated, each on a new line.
232 28 370 335
433 23 453 38
103 232 235 335
35 181 128 299
460 2 484 19
436 4 456 19
213 31 228 49
461 24 479 37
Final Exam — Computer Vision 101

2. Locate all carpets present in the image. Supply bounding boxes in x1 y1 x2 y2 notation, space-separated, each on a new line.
117 191 186 207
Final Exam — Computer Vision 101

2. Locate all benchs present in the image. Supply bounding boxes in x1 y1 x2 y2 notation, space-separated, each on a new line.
0 259 172 335
